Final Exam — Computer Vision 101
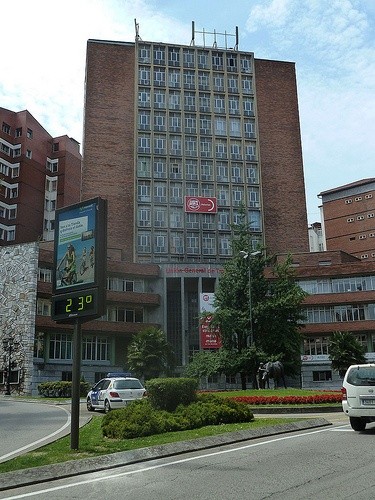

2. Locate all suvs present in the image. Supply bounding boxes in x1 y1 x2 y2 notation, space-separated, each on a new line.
342 363 375 431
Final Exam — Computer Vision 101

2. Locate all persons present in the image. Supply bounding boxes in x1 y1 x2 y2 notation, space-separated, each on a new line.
88 246 95 265
56 244 77 286
81 247 87 266
257 362 267 390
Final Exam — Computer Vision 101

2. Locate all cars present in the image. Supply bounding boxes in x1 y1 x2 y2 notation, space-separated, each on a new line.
85 372 149 413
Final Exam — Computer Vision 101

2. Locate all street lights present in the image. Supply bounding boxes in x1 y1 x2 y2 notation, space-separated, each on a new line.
241 248 261 346
3 337 19 395
